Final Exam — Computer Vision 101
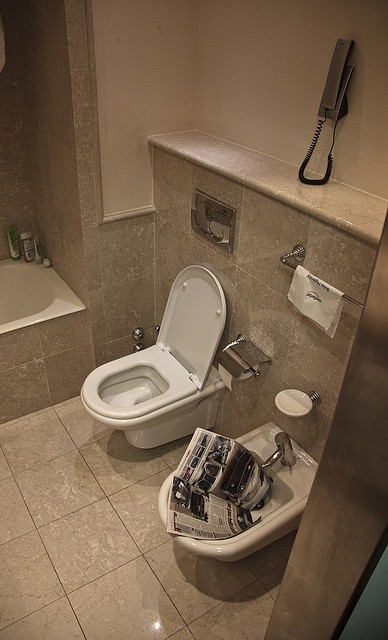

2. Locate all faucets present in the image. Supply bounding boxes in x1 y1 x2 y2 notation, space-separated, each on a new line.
260 431 298 471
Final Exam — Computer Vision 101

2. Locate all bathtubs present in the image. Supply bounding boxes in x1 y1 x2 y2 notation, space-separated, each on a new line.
0 251 97 334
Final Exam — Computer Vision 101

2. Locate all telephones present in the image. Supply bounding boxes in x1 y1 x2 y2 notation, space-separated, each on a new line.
297 38 356 186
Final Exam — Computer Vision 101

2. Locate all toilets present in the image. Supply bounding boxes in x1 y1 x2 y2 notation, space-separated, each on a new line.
80 265 227 448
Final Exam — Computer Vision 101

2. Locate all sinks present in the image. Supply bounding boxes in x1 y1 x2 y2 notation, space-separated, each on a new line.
159 422 321 560
